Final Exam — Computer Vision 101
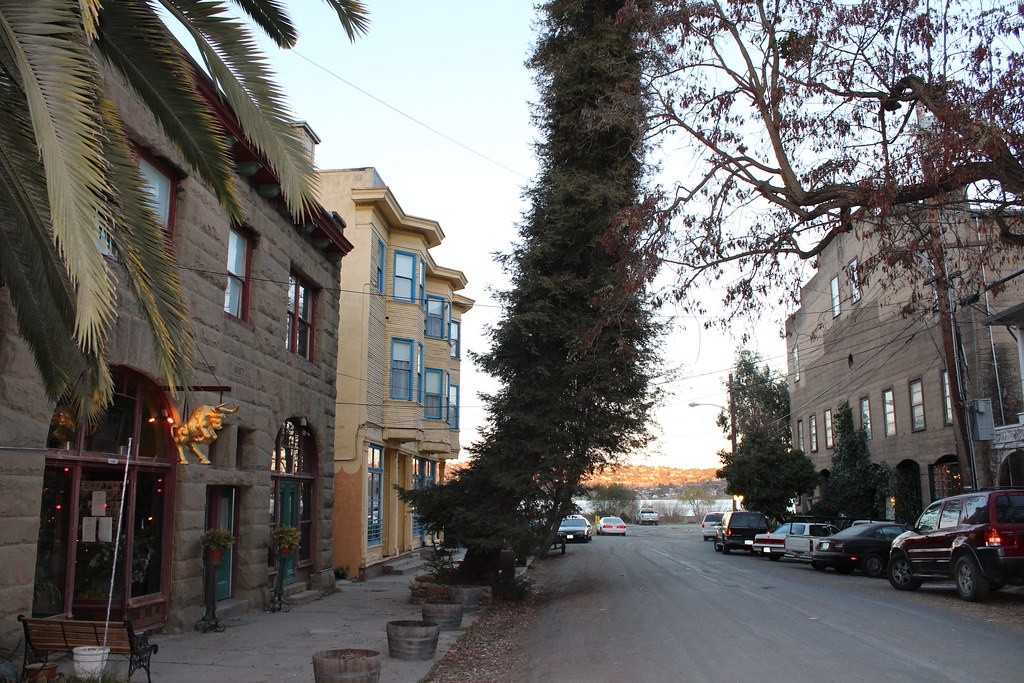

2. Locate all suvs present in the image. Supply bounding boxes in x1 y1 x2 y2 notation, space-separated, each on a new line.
887 486 1024 601
714 512 770 555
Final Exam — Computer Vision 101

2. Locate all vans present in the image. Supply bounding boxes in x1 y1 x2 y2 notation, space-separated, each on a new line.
702 512 726 541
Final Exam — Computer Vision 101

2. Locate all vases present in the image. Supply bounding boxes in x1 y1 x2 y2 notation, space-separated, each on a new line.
388 620 440 662
22 662 58 683
311 647 383 683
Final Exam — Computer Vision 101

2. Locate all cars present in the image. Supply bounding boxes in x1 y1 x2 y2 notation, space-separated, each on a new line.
753 523 842 564
813 522 910 578
556 518 592 543
597 517 627 536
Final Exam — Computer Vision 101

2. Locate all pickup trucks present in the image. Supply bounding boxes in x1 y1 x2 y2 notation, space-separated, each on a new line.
635 508 659 526
784 519 895 570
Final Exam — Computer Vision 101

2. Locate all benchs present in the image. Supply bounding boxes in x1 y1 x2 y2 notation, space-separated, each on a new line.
16 616 159 683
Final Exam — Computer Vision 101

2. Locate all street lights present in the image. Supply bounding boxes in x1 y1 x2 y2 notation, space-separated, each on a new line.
689 374 736 511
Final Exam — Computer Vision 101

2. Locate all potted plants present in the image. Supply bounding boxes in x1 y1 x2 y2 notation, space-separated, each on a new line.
199 528 236 565
273 524 302 556
420 584 465 631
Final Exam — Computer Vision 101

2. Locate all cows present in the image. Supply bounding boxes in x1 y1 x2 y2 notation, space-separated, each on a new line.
171 402 240 465
